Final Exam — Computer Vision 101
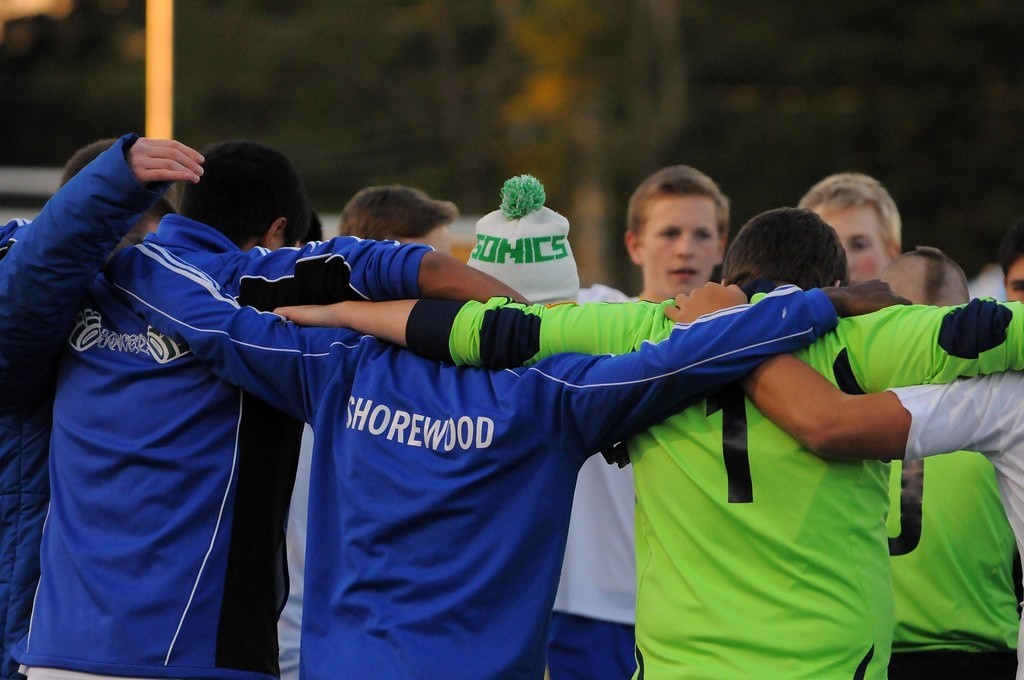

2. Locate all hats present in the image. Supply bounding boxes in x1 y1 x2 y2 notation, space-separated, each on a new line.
467 173 580 302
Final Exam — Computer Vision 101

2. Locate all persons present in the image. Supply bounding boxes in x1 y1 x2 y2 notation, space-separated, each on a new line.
270 206 1024 680
998 218 1024 303
795 173 901 285
275 183 458 679
0 134 206 680
873 246 1020 680
0 141 533 680
665 283 1024 680
547 163 729 680
0 173 912 680
296 212 323 248
